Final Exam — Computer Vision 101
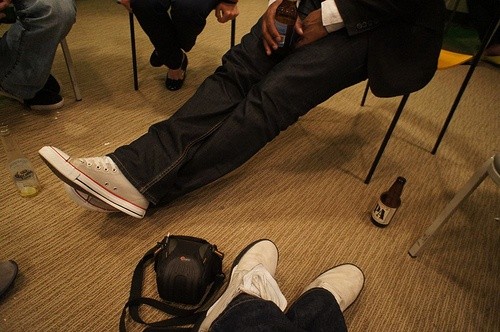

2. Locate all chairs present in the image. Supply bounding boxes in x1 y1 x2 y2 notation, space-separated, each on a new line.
361 0 500 186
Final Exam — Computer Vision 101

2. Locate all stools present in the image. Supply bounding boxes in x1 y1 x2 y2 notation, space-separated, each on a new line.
407 152 500 259
0 4 82 101
128 3 236 91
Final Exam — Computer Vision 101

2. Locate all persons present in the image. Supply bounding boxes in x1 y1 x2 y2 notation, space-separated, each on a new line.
192 238 366 332
118 0 240 91
39 0 446 220
0 0 77 110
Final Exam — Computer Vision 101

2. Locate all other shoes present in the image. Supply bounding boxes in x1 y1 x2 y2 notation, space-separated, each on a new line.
199 239 281 332
300 262 367 315
37 143 150 219
0 72 65 111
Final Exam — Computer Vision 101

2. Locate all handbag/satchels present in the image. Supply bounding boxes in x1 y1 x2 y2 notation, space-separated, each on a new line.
119 230 227 332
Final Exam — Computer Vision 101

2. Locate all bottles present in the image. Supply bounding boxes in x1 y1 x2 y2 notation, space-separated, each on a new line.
371 176 406 228
0 0 17 24
1 122 41 197
271 0 299 58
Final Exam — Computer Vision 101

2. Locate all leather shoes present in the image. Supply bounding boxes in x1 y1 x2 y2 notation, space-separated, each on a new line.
149 49 164 67
165 52 189 91
